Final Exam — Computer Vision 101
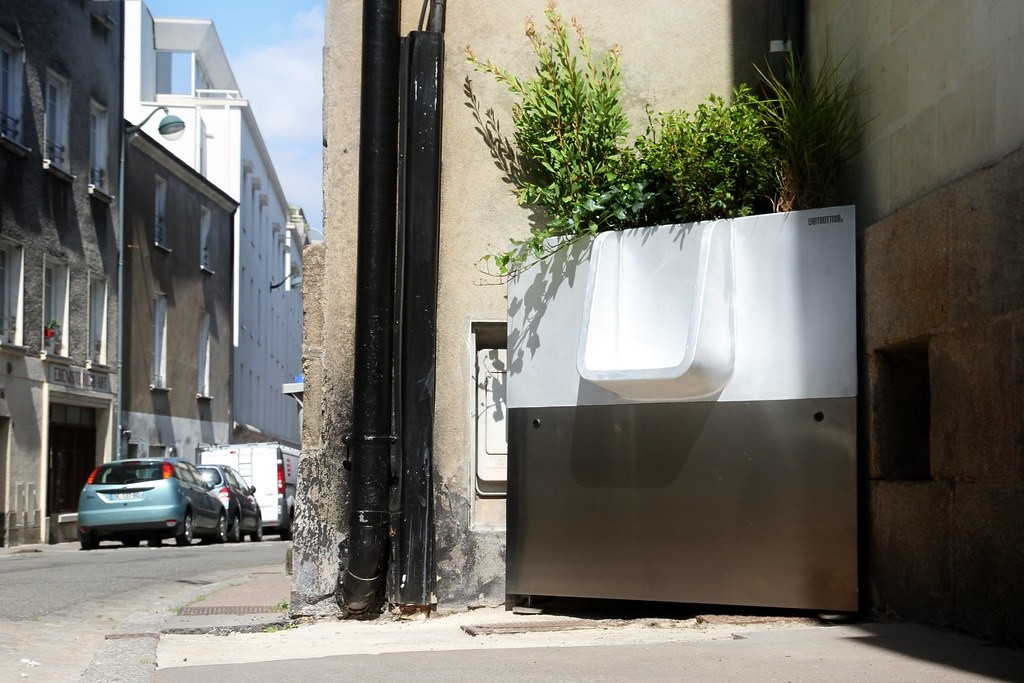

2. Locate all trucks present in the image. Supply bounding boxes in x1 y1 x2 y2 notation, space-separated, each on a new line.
197 441 302 540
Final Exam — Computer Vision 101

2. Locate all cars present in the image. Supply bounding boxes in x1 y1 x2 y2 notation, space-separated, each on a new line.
193 464 262 543
77 456 228 551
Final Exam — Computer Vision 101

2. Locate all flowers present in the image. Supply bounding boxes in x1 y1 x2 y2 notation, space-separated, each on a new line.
45 321 62 341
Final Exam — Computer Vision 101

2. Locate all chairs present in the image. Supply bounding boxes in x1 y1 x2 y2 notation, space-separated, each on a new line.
106 472 125 484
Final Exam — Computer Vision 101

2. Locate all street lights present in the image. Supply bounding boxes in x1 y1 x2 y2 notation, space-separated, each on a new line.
115 103 185 461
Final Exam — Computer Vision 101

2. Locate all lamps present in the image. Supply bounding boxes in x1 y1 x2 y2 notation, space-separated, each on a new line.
124 105 186 140
269 272 302 293
